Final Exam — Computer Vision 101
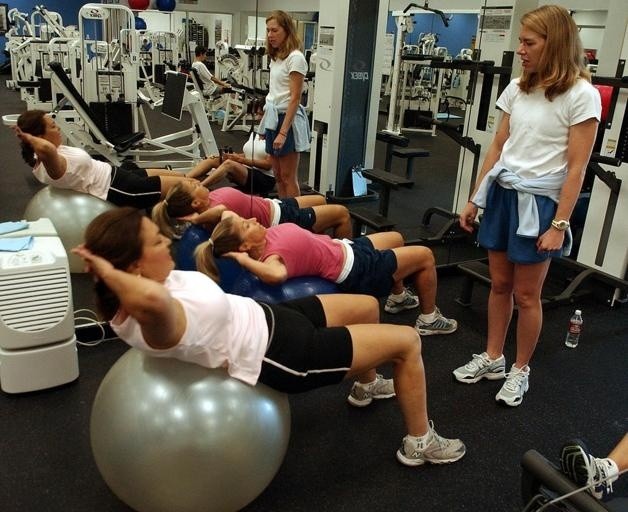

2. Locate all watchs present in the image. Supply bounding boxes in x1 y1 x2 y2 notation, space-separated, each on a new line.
551 218 571 230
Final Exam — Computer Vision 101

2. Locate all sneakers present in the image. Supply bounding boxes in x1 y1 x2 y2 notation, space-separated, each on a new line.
558 438 617 503
384 286 420 314
494 362 531 407
414 305 458 336
452 352 506 384
396 420 467 466
348 374 396 407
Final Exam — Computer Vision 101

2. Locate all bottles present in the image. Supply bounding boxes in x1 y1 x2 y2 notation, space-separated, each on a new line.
565 309 583 349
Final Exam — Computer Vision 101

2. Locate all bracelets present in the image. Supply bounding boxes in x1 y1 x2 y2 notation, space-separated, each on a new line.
279 131 288 138
467 200 472 203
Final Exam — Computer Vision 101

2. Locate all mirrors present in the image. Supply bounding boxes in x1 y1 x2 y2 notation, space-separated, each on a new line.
0 0 486 324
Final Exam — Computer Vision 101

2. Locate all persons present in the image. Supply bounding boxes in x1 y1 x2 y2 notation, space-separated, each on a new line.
558 429 628 501
451 4 604 409
186 97 275 196
192 44 241 113
257 9 312 199
150 179 353 240
192 209 458 338
11 109 195 209
141 39 152 51
70 206 466 469
